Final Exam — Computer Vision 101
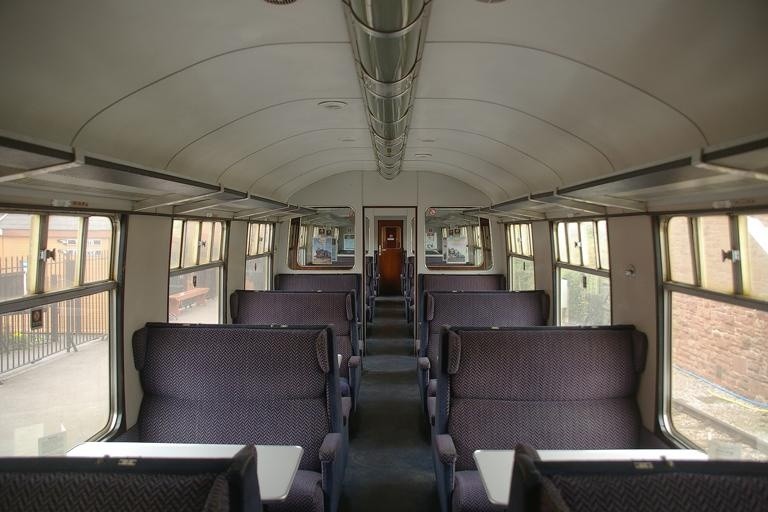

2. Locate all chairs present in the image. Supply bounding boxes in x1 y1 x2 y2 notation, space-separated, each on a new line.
400 249 767 511
2 250 377 512
